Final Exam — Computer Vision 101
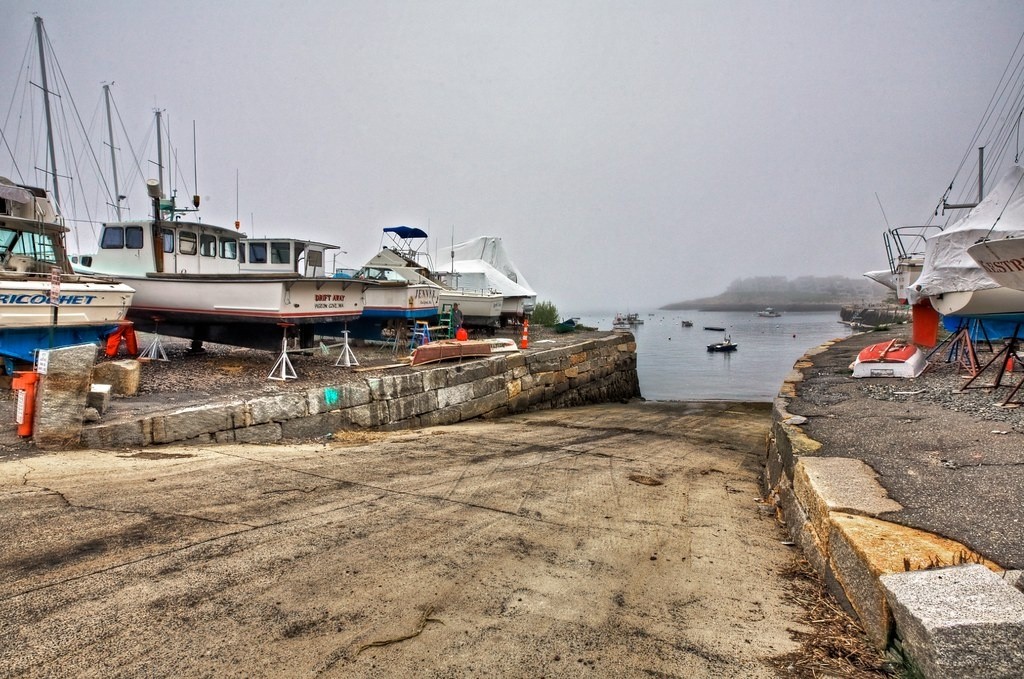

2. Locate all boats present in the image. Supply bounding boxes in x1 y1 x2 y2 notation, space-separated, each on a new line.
703 326 726 331
706 325 738 352
476 338 521 353
0 129 137 372
850 337 929 379
0 7 539 357
410 336 494 367
864 35 1024 345
757 306 782 318
611 311 645 329
681 319 694 327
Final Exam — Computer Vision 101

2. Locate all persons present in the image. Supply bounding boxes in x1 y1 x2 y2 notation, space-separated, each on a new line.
448 303 463 338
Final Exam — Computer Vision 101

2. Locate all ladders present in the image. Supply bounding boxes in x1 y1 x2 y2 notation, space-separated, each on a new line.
410 320 430 352
435 304 455 339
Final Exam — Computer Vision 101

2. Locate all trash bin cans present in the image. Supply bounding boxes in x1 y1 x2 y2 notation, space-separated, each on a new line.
9 370 39 439
